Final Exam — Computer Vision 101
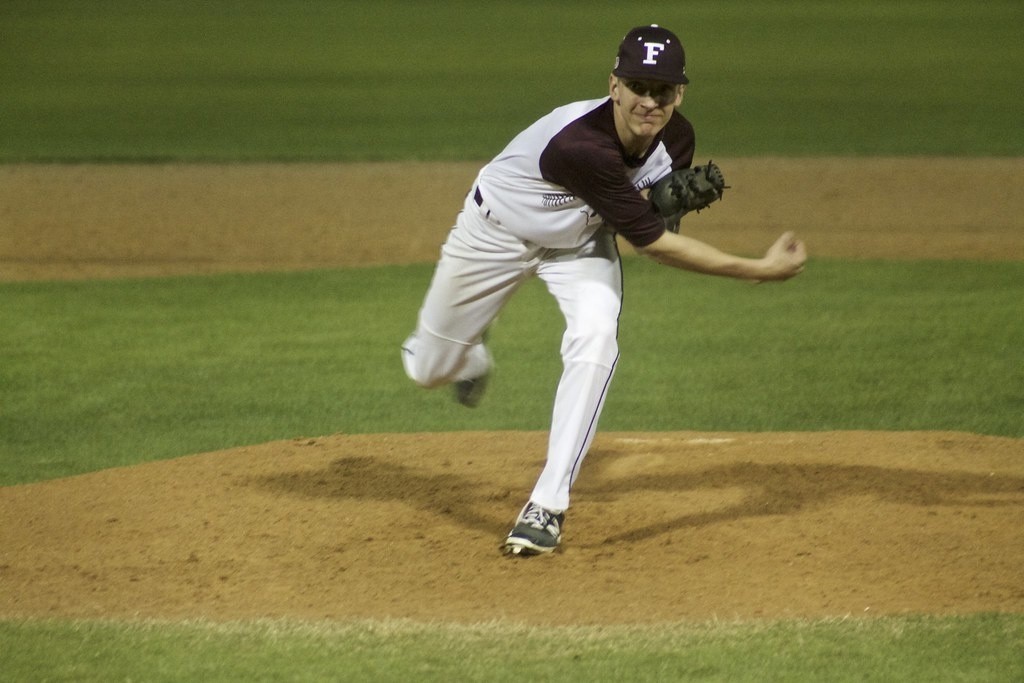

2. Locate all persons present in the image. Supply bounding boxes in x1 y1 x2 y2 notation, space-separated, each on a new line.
402 25 805 556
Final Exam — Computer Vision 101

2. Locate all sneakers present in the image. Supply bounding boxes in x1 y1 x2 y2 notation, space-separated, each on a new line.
455 319 499 407
501 501 564 555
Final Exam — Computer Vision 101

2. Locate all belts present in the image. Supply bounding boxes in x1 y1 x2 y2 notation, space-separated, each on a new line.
473 187 490 216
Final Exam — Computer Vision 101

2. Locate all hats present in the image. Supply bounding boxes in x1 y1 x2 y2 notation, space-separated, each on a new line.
612 23 690 85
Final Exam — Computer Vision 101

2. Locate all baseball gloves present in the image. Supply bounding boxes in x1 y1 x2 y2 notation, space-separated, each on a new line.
647 158 732 234
